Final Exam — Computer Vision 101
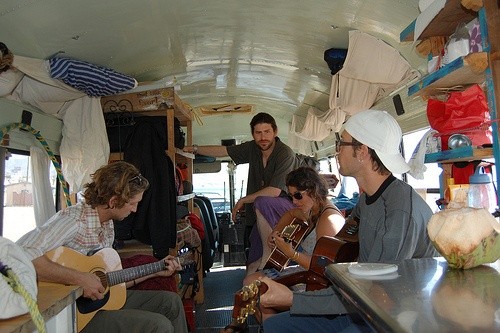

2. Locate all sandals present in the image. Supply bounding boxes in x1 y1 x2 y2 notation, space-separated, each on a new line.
223 319 250 333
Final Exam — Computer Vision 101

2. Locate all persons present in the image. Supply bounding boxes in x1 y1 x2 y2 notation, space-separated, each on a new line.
259 110 440 333
243 167 345 321
184 113 299 275
17 162 187 333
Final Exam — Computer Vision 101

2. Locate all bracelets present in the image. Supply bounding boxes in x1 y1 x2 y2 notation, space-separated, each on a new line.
134 280 136 286
193 145 199 153
291 252 299 261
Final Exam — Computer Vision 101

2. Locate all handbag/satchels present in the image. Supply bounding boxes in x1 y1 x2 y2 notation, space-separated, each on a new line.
426 84 492 135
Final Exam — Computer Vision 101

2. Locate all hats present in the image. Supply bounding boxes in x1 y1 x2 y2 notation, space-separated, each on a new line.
342 110 412 174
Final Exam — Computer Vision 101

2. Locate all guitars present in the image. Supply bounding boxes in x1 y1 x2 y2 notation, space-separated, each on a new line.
263 216 310 273
231 236 358 324
37 245 195 333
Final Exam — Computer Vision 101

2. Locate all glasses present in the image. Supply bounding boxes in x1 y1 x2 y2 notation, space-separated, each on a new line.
287 189 309 202
128 173 143 186
335 138 363 152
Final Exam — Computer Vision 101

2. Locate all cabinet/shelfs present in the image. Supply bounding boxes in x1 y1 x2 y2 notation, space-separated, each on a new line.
100 86 204 307
0 282 83 333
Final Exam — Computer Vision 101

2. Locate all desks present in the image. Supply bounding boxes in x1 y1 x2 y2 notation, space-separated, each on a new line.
323 256 500 333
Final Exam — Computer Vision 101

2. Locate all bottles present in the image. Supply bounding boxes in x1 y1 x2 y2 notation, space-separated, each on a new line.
468 165 498 212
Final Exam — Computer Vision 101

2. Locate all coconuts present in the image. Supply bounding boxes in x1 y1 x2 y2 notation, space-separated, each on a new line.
427 206 500 269
